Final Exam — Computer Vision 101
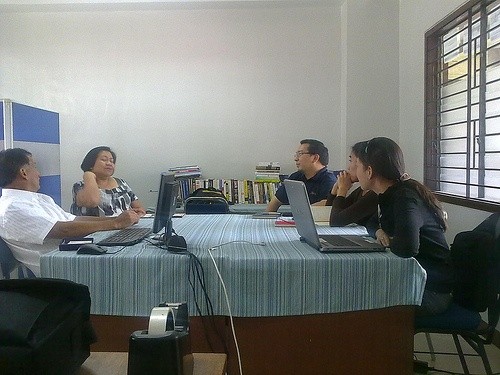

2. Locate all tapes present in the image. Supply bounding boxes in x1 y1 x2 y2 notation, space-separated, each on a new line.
147 306 175 336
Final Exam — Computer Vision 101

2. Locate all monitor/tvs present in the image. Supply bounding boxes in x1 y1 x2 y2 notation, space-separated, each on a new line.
150 170 180 246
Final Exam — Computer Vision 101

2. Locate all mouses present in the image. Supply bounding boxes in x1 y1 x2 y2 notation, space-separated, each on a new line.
75 243 107 255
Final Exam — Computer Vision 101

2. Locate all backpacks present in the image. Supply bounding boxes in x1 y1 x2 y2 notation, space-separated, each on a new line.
184 187 230 215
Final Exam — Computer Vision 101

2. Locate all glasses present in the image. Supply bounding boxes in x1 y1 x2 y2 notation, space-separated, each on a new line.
294 151 313 158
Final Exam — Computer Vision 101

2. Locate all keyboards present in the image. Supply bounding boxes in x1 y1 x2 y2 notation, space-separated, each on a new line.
96 228 153 246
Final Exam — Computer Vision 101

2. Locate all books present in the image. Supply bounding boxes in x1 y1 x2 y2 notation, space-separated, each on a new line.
161 161 282 204
275 221 296 226
253 212 281 219
277 205 293 217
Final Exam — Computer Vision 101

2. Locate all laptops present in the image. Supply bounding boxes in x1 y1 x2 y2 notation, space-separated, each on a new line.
283 179 386 252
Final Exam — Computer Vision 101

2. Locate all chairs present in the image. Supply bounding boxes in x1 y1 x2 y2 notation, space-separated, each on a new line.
413 229 500 375
0 236 23 280
182 197 229 215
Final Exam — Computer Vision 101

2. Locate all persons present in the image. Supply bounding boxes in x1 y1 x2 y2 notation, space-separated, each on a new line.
0 148 139 278
71 145 146 217
265 139 337 212
325 142 380 229
356 137 455 318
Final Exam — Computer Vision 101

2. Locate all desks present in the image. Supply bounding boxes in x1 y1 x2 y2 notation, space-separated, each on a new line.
40 213 427 375
